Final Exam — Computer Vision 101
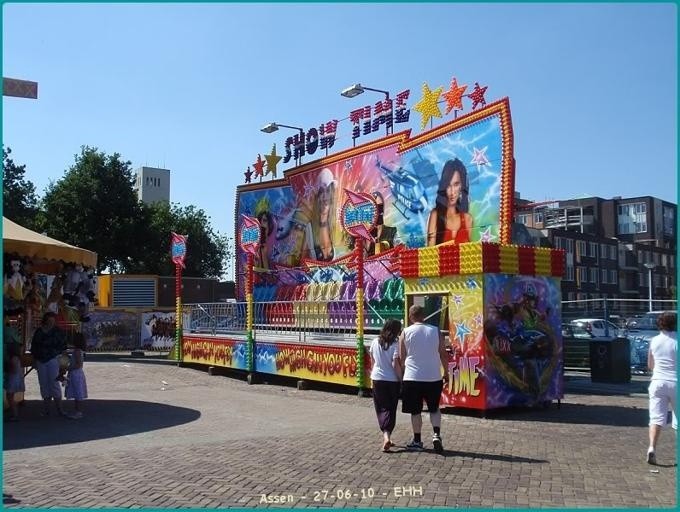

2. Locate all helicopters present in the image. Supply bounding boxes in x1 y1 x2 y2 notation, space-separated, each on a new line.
374 155 428 220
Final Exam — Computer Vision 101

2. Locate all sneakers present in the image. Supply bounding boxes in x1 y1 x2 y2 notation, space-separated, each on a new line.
648 449 656 465
407 436 443 452
43 409 85 420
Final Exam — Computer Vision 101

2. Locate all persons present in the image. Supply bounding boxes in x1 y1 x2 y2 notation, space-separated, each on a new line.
3 342 26 421
30 311 68 417
647 313 677 464
513 282 553 394
349 191 397 256
64 332 88 419
251 210 276 275
369 320 403 452
428 157 472 247
398 304 448 453
294 167 339 267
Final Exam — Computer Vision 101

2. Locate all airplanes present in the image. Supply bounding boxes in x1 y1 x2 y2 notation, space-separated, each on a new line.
265 206 306 241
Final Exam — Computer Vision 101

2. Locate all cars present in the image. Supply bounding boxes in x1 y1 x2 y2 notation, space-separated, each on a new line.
560 310 678 375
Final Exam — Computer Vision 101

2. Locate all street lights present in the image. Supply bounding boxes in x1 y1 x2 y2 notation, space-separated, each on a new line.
644 263 656 312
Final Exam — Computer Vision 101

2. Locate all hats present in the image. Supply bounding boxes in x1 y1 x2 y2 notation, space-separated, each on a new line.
315 167 338 190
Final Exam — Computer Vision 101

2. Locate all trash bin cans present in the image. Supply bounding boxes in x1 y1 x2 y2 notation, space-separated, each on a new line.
589 336 631 384
